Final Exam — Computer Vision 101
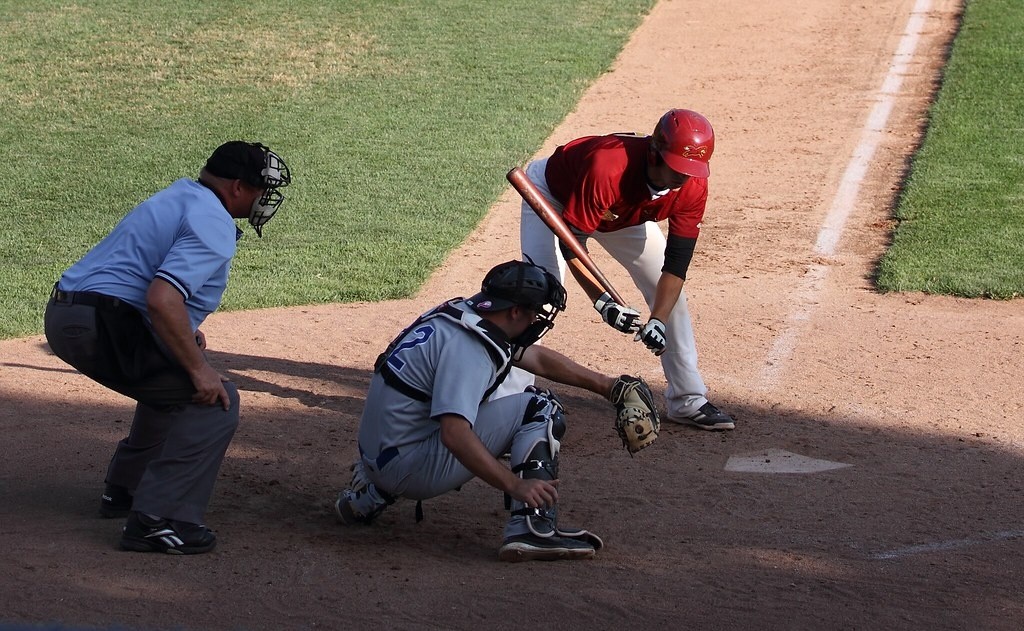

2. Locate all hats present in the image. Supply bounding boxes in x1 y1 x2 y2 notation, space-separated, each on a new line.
205 141 265 187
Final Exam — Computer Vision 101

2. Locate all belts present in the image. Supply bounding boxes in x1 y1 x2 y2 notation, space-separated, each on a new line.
50 287 129 308
358 440 399 471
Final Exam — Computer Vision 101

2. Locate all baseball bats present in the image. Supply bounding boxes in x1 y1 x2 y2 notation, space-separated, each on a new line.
507 168 667 357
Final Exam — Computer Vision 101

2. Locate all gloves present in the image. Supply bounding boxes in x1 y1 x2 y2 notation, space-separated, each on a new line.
633 317 667 352
593 291 642 334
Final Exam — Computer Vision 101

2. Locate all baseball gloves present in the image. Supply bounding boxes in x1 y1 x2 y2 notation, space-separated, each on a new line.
609 373 661 455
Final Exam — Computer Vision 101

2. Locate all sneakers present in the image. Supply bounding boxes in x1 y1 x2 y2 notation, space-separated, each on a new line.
120 509 216 554
335 488 362 526
99 489 134 518
667 401 735 430
500 532 595 561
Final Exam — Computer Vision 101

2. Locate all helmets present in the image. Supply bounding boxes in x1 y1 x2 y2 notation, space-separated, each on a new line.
464 259 547 312
653 108 714 179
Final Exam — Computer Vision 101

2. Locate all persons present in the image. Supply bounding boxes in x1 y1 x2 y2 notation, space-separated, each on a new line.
335 262 659 564
45 141 290 554
519 107 735 430
